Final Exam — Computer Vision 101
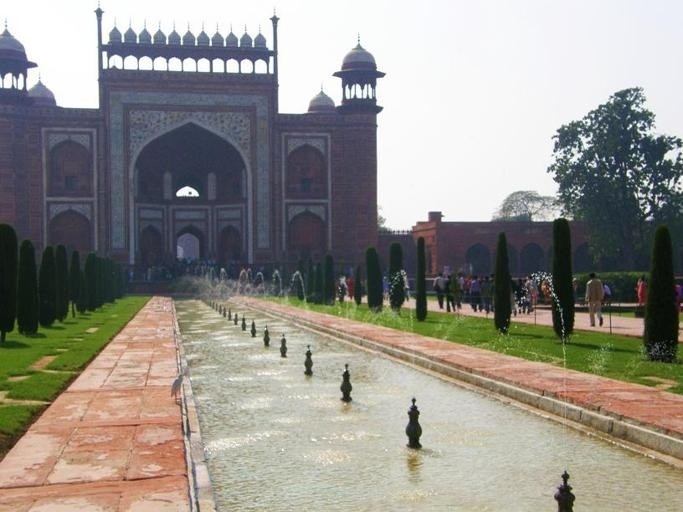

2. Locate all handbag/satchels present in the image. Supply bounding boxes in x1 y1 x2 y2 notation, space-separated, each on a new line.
433 284 442 293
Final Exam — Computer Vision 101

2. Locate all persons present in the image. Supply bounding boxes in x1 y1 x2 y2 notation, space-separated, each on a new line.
432 271 539 313
345 272 355 300
399 268 410 303
632 275 647 307
603 284 611 298
584 272 604 328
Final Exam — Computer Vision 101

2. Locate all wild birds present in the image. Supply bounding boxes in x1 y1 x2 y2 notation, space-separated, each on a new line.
169 373 185 403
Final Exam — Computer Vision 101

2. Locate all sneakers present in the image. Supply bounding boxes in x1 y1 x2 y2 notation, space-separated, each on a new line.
591 324 595 326
599 317 603 327
